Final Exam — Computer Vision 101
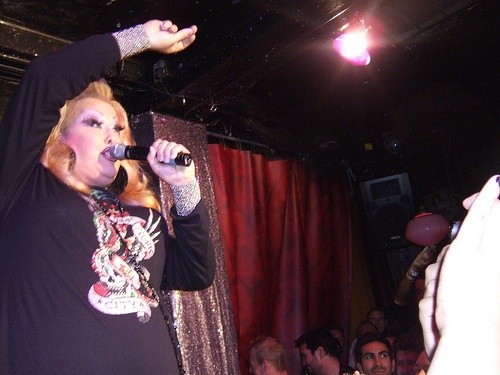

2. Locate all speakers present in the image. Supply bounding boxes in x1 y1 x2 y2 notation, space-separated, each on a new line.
359 172 417 252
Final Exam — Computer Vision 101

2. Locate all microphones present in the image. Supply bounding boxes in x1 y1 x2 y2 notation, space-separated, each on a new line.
110 142 192 166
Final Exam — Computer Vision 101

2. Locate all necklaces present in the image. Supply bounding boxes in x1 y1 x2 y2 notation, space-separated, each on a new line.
91 191 186 375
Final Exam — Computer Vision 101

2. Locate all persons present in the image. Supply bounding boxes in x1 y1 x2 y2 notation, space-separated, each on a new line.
325 246 437 375
248 336 288 375
294 328 355 375
419 175 499 375
0 20 216 375
